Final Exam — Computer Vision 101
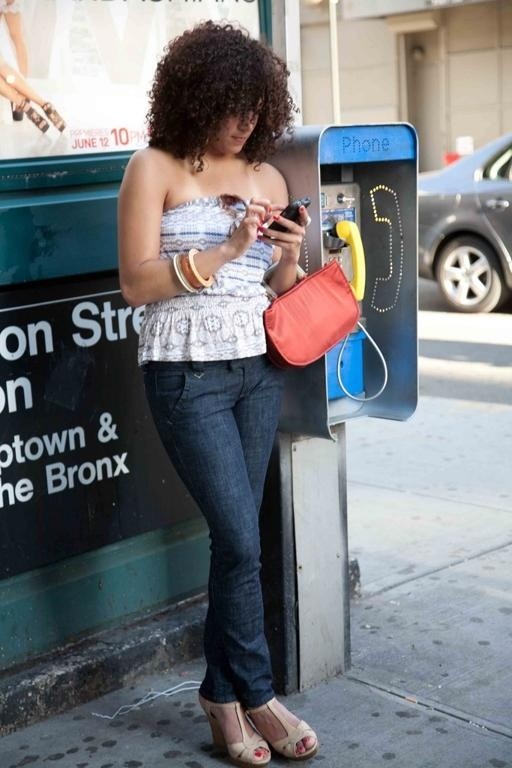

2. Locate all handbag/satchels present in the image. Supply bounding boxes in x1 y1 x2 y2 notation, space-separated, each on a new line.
261 257 360 369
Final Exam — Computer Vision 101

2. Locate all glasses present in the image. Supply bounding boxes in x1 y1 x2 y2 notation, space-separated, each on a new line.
219 194 248 214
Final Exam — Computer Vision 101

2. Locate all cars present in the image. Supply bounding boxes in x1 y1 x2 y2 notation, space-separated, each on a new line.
415 133 512 314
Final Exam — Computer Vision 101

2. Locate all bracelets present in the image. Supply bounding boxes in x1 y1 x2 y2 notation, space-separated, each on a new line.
172 247 214 296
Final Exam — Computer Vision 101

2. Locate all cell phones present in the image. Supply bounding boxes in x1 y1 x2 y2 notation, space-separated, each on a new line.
262 197 312 239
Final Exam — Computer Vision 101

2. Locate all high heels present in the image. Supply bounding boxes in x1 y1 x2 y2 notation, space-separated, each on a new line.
197 692 272 766
247 696 319 762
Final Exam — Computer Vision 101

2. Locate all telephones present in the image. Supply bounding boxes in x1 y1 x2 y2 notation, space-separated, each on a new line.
320 182 368 334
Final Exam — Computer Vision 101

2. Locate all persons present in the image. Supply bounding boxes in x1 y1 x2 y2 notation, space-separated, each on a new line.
114 20 320 766
0 1 67 135
